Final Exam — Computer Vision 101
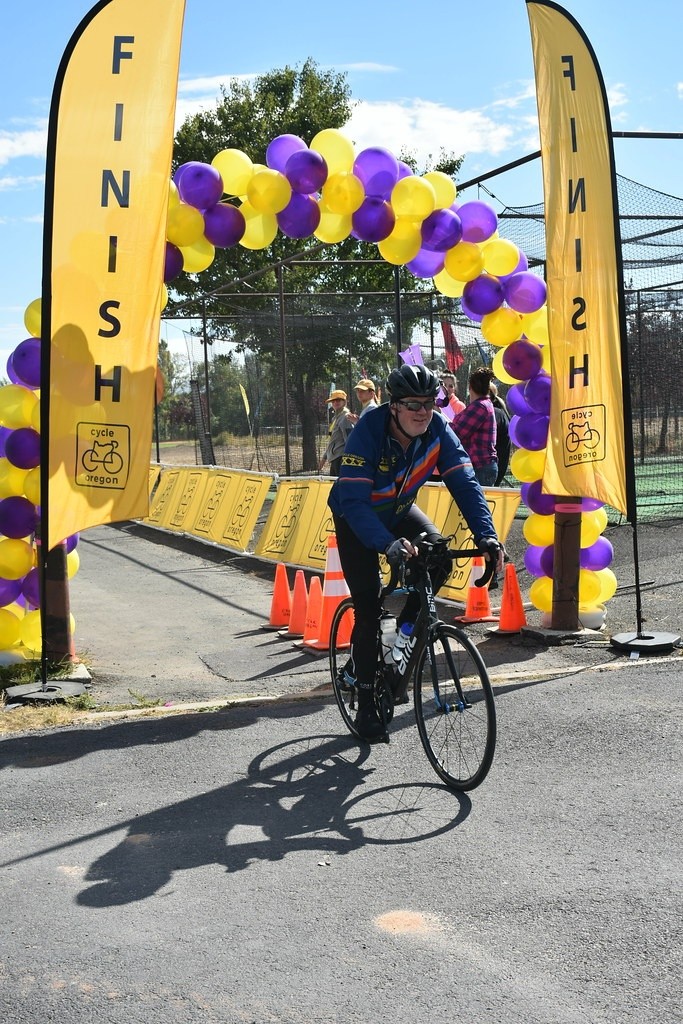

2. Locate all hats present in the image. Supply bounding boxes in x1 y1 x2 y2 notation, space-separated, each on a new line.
326 390 347 402
354 379 376 391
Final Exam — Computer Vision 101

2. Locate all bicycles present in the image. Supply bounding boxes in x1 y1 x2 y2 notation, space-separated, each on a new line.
328 531 510 791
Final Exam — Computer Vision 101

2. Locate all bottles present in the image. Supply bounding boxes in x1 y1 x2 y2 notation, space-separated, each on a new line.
393 623 414 660
380 610 398 664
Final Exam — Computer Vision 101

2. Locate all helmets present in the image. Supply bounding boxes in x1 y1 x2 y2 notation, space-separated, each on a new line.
386 364 440 398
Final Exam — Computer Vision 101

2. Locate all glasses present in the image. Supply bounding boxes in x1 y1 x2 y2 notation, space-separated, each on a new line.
398 400 434 411
332 399 344 403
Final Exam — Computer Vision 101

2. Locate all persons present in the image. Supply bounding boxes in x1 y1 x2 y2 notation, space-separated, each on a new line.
448 367 498 486
346 379 378 425
490 382 510 486
326 364 507 744
317 390 354 476
428 369 467 483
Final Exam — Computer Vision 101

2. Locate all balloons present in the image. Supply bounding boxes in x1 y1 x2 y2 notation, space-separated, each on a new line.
403 199 530 299
460 274 552 385
309 128 457 265
504 379 608 515
162 132 328 311
0 455 80 581
523 508 617 610
0 299 41 469
0 567 75 650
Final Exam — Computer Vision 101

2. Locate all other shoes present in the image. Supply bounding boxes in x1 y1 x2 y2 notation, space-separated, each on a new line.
396 619 414 637
354 705 389 744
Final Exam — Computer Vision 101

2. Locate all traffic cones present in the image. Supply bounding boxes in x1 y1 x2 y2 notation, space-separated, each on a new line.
260 562 291 630
305 534 355 650
454 556 500 624
293 576 323 648
486 562 527 636
278 570 309 639
